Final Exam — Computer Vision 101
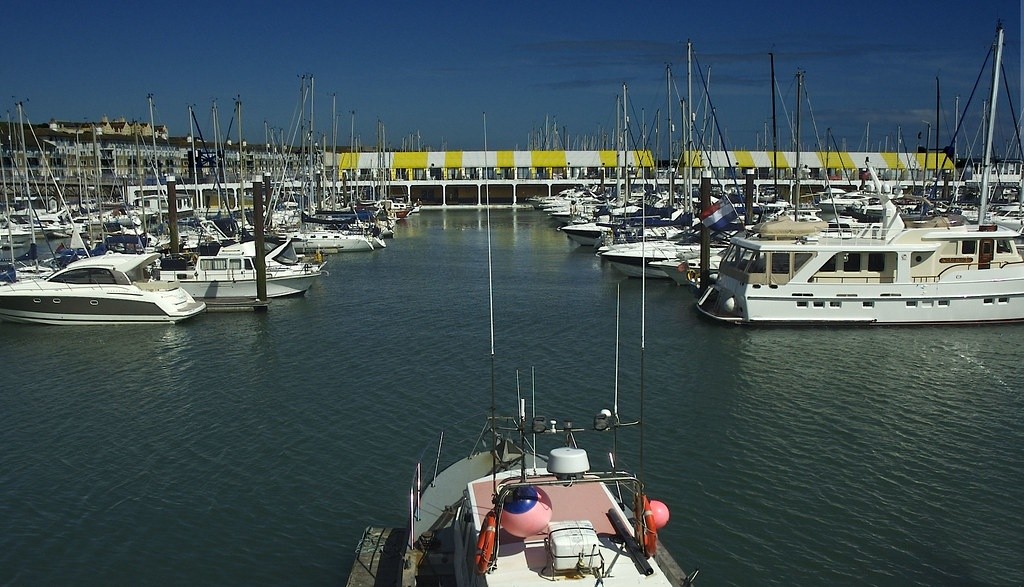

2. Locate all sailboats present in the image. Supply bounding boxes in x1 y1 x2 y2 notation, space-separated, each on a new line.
0 71 419 325
527 18 1024 327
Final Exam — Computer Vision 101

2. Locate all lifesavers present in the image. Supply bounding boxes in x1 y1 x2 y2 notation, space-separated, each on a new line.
634 492 658 558
473 511 499 574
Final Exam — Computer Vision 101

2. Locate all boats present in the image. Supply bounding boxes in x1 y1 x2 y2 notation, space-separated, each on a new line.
399 80 699 587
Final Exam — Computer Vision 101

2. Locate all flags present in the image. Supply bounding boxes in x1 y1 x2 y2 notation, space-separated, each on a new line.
698 193 739 233
